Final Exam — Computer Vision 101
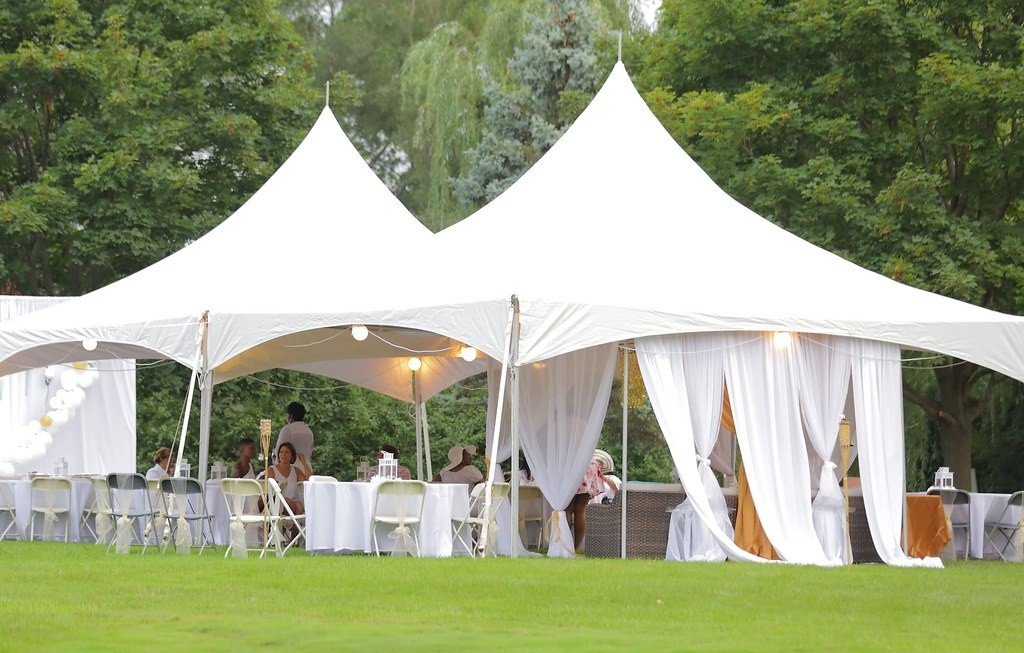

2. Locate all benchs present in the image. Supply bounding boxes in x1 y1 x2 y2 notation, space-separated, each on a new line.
584 481 883 560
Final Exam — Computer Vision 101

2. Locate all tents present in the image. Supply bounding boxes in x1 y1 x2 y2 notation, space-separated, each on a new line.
1 26 1024 570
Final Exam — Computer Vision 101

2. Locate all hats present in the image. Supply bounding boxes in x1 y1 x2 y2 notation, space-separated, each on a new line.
444 445 476 472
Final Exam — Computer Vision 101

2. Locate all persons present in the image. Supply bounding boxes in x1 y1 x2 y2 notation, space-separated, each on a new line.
589 449 621 505
146 446 174 481
232 437 265 513
510 455 536 486
564 454 603 551
377 444 411 480
268 442 312 547
432 446 486 495
272 401 314 477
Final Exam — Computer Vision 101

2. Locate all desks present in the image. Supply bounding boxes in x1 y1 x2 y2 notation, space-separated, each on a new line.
111 485 258 546
3 478 93 543
302 481 472 558
908 492 1023 560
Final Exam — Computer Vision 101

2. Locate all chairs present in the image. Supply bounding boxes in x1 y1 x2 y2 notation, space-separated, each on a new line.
927 489 971 562
451 483 511 559
370 481 426 559
518 486 545 551
983 491 1024 563
0 472 338 560
